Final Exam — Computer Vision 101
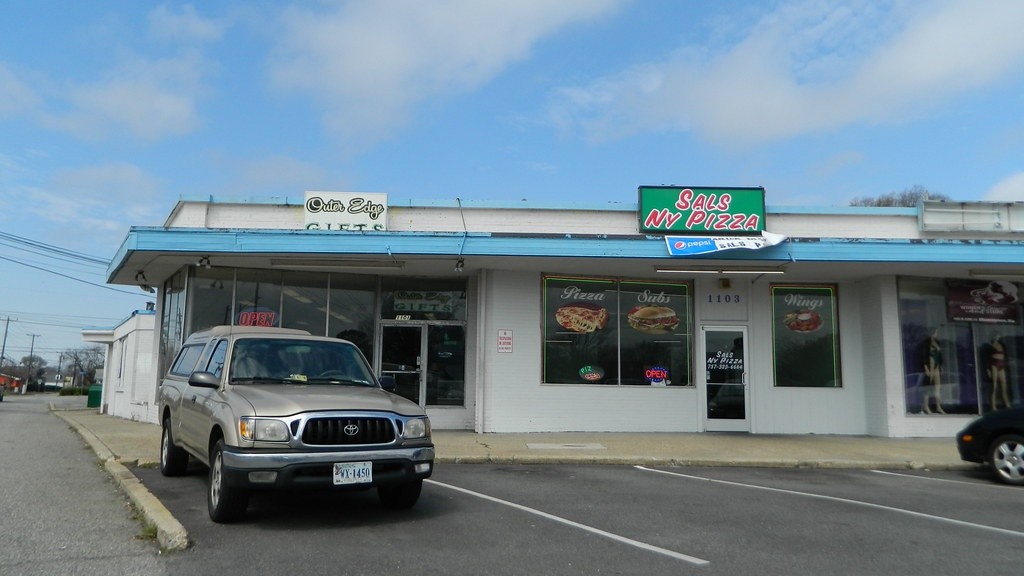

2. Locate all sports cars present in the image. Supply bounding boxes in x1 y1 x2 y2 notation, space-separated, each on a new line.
956 407 1024 486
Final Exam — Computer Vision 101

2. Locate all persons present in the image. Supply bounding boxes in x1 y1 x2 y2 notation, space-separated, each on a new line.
914 327 948 414
230 343 270 377
986 330 1012 411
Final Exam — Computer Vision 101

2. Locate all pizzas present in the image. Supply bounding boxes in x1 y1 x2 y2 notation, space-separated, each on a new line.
557 307 606 332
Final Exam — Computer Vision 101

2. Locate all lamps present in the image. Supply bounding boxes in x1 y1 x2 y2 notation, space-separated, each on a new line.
269 259 407 269
655 266 785 274
454 258 465 272
195 256 211 269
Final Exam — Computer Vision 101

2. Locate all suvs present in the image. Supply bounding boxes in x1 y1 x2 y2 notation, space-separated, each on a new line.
158 326 435 522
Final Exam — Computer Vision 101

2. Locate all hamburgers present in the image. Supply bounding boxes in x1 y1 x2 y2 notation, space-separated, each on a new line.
628 306 678 332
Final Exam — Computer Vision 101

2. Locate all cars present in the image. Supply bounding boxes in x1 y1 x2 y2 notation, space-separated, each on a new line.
906 372 973 408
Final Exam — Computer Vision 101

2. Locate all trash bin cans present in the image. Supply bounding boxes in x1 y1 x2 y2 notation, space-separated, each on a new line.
88 384 102 407
